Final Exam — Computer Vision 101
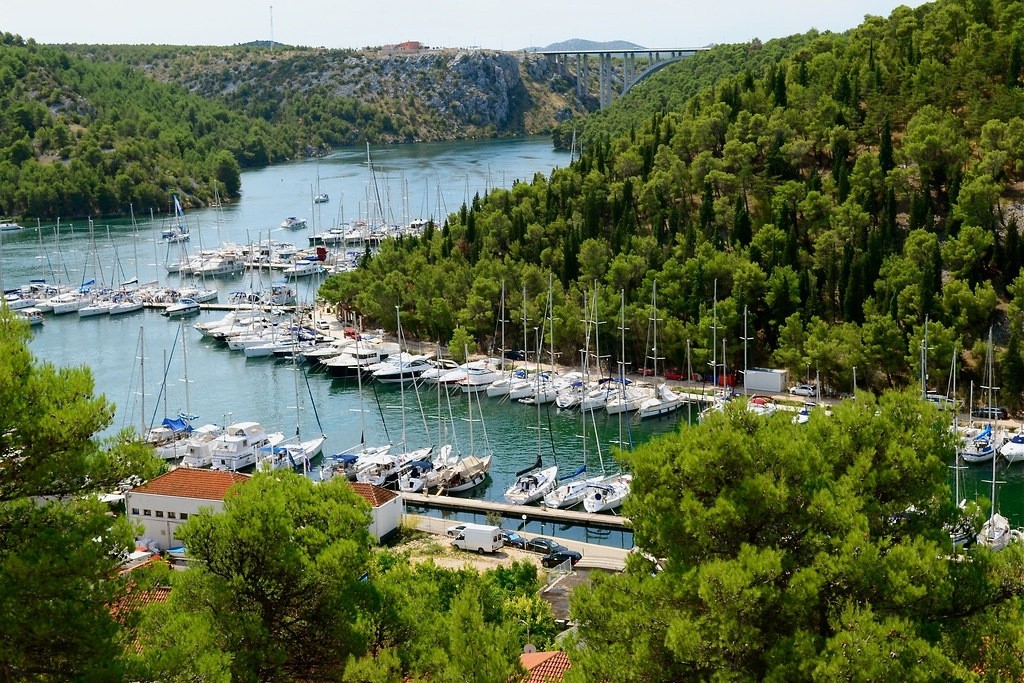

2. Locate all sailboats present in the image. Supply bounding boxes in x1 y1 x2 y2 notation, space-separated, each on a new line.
791 369 836 426
633 279 689 418
692 277 777 425
892 320 1024 557
1 140 653 513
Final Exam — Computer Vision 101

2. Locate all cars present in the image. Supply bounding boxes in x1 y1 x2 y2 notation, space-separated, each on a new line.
527 537 568 554
970 406 1010 421
638 366 656 376
540 551 582 568
446 523 473 538
501 530 528 549
316 320 331 330
664 368 689 381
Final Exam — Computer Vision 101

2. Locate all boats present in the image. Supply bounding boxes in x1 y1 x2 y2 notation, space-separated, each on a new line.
0 222 25 232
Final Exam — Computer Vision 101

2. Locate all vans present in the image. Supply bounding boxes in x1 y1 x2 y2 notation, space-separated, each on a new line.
448 524 504 555
344 328 361 341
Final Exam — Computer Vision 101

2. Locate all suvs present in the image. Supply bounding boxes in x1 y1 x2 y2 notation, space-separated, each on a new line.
790 385 817 398
